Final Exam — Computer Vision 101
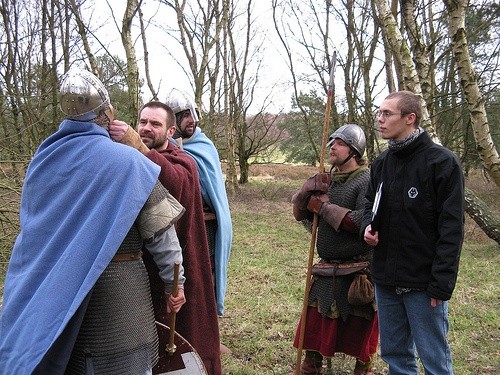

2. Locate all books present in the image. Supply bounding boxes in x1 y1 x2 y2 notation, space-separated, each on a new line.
371 181 382 234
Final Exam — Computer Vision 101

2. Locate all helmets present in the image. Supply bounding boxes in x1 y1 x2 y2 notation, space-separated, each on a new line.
165 87 199 123
326 124 366 157
59 70 110 121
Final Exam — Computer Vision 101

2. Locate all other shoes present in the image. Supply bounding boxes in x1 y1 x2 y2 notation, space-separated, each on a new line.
354 357 375 375
301 351 323 375
220 344 233 355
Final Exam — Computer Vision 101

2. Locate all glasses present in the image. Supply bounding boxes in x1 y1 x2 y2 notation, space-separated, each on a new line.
376 112 411 119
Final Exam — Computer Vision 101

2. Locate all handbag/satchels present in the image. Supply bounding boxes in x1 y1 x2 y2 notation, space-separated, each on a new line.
348 273 375 305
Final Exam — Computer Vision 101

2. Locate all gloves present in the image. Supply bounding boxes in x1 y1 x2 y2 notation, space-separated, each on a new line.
293 173 332 221
307 192 352 233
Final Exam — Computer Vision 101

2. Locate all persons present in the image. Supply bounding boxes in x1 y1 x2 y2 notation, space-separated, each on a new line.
291 124 378 375
109 102 221 375
362 91 464 375
0 68 186 375
165 88 232 353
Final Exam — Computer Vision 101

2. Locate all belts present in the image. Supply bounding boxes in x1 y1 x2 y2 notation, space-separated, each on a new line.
112 251 144 261
